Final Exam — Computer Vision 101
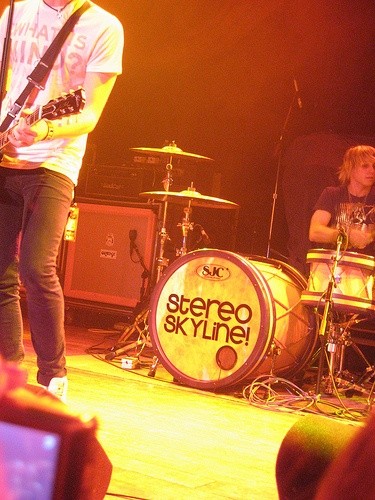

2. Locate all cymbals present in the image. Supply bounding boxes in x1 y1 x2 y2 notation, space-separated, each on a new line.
139 190 240 210
129 146 215 163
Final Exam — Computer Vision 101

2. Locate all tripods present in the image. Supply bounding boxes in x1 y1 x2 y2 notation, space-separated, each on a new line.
107 159 200 378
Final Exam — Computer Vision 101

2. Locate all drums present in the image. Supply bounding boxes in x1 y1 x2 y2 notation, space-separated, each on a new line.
149 247 318 390
300 248 375 315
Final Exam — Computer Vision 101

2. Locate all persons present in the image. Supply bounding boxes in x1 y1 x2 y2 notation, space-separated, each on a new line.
0 0 127 405
308 145 375 371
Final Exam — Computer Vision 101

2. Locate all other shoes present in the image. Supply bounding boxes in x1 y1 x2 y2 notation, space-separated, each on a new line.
36 376 71 400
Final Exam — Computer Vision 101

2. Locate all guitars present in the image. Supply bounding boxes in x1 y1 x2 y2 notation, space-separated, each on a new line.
0 85 88 166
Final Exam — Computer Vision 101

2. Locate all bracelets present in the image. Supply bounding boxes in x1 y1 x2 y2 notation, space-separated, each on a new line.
42 118 52 143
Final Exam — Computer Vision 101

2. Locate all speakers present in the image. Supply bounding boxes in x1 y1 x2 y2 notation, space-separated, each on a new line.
66 202 155 308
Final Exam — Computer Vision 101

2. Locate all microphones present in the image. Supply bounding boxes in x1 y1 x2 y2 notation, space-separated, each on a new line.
294 81 302 108
200 228 210 246
